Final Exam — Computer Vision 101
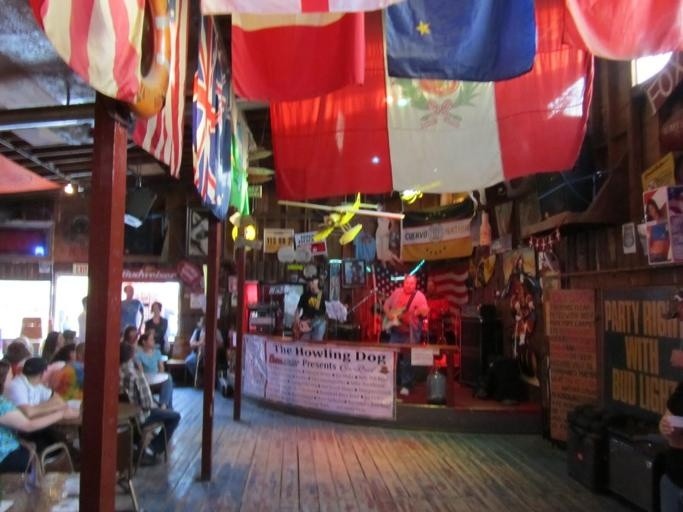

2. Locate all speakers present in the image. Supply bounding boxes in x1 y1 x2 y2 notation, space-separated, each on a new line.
124 197 169 257
458 318 504 388
535 130 592 220
608 412 669 512
565 402 636 493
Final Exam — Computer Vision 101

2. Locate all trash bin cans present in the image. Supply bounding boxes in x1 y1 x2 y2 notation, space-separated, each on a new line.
566 398 683 512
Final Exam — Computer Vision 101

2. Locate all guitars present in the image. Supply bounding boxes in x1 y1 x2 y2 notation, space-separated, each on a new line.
382 307 427 333
291 313 327 341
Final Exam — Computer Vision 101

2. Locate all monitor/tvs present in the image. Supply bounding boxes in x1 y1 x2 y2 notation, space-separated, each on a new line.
0 227 46 254
340 258 368 290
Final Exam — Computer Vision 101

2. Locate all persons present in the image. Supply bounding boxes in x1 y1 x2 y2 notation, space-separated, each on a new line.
294 275 328 341
661 371 683 512
185 317 229 385
384 276 429 396
0 286 181 470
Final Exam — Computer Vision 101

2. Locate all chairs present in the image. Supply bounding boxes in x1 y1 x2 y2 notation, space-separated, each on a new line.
0 390 168 511
166 335 191 384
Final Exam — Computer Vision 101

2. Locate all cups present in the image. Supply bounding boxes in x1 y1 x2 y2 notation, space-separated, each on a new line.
66 399 81 409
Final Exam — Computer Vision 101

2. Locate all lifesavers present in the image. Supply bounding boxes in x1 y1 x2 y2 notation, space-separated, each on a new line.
122 0 170 120
